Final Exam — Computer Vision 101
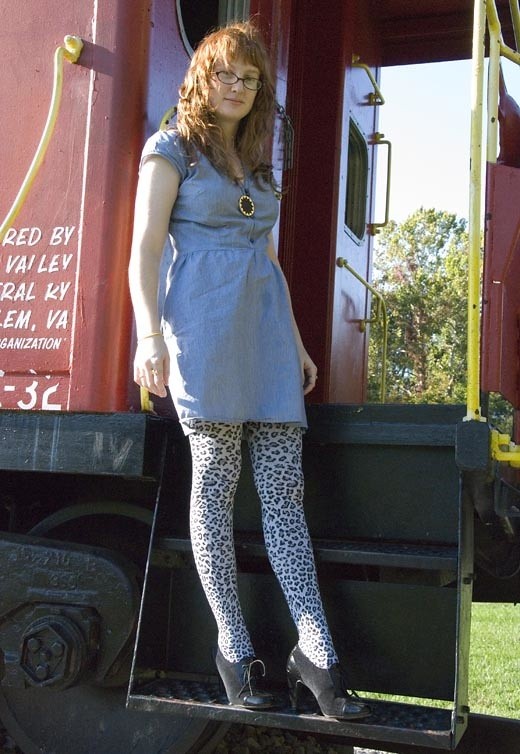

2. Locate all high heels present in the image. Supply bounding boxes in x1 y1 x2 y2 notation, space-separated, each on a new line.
286 642 373 719
216 648 277 710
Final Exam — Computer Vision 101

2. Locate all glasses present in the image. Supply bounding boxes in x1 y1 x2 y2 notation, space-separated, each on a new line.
210 71 263 91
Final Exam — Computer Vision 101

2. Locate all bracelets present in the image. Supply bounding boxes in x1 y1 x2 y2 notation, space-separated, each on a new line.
137 332 163 344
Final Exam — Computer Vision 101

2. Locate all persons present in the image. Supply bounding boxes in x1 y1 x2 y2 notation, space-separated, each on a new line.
129 24 373 718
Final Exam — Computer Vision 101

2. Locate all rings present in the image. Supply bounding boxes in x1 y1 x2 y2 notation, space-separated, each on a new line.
151 370 163 376
139 375 145 378
308 376 318 379
